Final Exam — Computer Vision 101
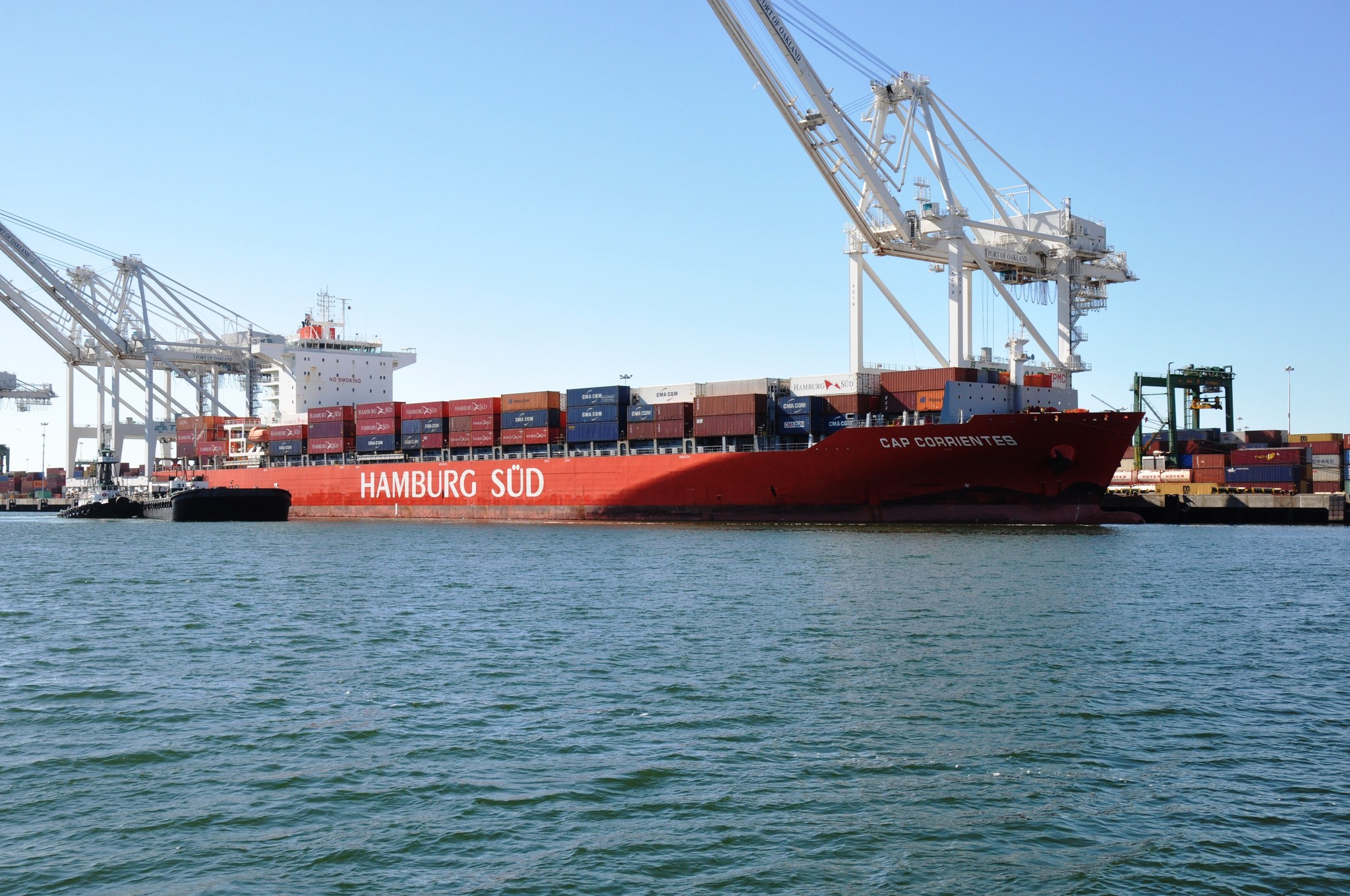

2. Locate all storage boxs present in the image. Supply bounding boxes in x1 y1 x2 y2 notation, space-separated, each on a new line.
500 356 1052 445
1111 428 1350 495
268 397 498 459
176 415 260 466
298 326 336 340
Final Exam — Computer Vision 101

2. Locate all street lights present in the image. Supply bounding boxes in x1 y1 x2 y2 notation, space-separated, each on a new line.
79 442 83 464
40 423 48 491
26 459 29 472
1236 417 1249 431
1284 366 1294 434
620 374 633 386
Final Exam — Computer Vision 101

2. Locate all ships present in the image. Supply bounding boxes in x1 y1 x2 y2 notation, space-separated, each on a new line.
152 315 1146 524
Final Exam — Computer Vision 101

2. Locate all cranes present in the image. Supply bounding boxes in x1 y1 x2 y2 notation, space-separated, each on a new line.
0 209 285 495
706 0 1140 389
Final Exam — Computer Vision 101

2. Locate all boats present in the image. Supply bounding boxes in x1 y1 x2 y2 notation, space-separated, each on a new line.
138 468 292 522
57 424 143 519
